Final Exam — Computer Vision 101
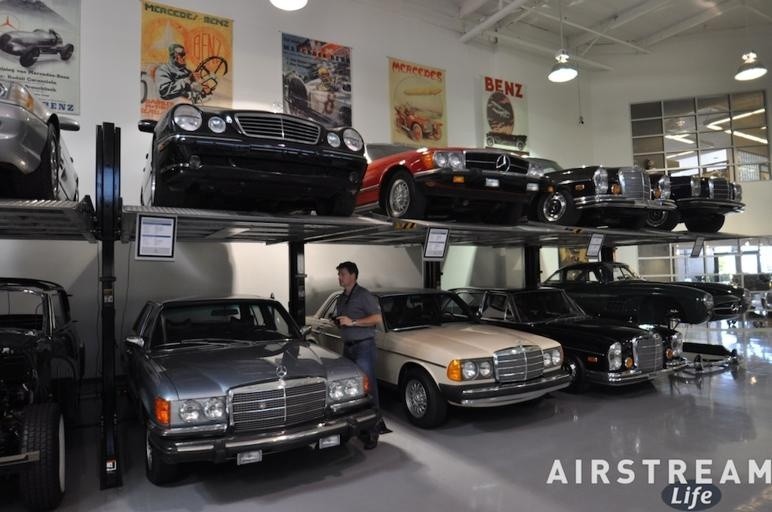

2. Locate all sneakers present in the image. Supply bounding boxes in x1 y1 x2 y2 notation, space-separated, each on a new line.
364 432 379 449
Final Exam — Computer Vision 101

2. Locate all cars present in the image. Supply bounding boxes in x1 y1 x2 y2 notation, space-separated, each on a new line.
524 158 678 230
138 99 368 219
299 287 573 429
0 77 79 199
354 142 543 221
116 292 383 487
650 170 743 233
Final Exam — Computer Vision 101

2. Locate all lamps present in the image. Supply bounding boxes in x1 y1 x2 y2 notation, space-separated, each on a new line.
731 11 768 87
542 11 582 87
268 0 308 11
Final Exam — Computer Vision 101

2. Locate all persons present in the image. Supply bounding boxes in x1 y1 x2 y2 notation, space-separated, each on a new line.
154 43 203 101
332 261 383 449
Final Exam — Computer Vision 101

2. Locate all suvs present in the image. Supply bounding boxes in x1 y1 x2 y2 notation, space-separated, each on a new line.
0 278 86 512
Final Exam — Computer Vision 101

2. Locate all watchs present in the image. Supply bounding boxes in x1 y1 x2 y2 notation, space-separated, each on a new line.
351 318 357 327
